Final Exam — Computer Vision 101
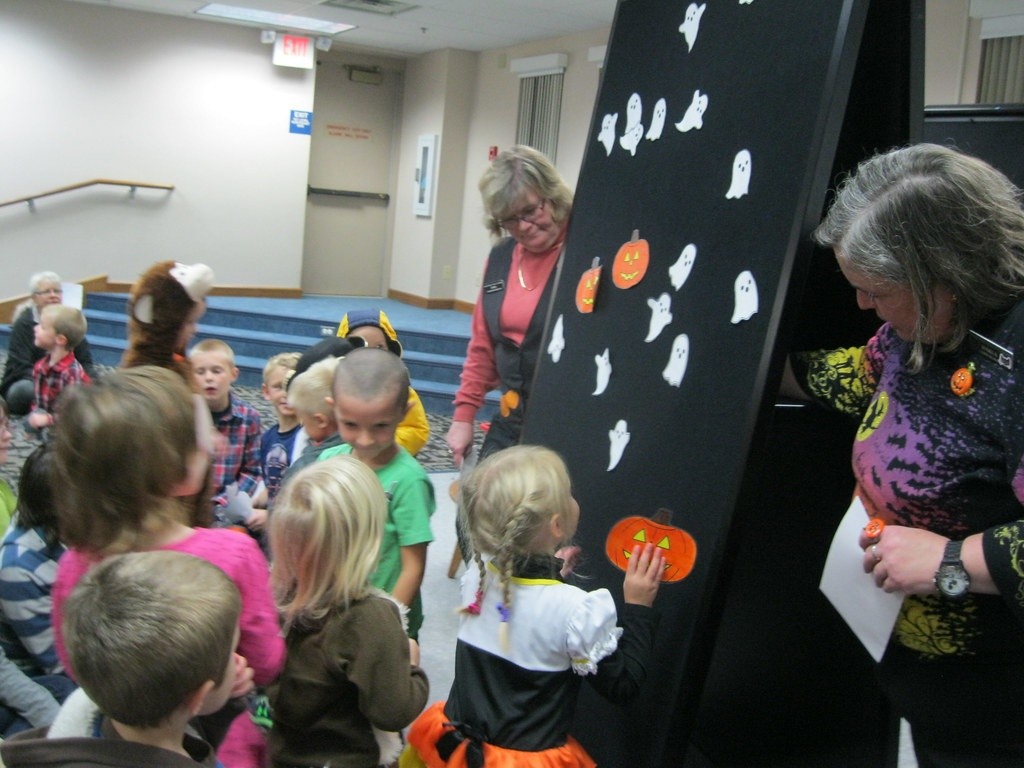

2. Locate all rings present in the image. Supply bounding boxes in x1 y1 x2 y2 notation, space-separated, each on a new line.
872 544 880 561
863 520 883 542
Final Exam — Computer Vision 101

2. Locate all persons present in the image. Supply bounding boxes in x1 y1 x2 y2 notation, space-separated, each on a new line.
0 260 436 767
779 144 1024 768
398 445 667 768
445 145 574 458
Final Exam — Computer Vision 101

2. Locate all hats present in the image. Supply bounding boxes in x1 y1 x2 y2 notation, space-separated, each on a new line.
285 336 353 391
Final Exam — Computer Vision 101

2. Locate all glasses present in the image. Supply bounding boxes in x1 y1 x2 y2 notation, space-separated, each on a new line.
496 198 545 229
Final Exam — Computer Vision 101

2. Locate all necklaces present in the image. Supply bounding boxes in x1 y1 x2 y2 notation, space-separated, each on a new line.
518 252 540 291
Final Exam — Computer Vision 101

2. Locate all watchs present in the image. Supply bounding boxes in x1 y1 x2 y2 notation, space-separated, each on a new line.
933 540 971 600
